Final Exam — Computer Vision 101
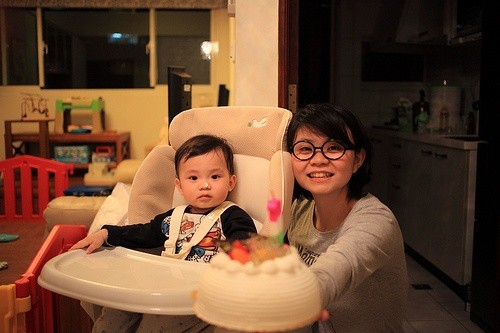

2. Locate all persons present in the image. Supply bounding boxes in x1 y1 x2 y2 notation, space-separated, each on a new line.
287 106 409 333
68 134 257 333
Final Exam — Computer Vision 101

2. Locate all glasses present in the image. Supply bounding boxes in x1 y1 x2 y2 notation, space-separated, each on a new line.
289 138 358 161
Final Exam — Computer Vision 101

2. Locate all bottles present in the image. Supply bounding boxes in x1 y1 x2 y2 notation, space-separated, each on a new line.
413 91 430 127
440 106 449 126
466 112 476 135
267 199 283 249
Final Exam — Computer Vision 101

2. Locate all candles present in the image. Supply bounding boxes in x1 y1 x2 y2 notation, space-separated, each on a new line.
267 189 283 245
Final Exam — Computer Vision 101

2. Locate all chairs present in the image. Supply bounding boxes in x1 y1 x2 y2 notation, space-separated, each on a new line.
126 106 295 246
42 158 140 226
0 155 68 226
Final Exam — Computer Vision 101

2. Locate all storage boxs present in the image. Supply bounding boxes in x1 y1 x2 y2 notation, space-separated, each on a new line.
53 145 88 163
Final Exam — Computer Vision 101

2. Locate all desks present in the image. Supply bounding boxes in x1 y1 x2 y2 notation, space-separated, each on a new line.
0 221 49 286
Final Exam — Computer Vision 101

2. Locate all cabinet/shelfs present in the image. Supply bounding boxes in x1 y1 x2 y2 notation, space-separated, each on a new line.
4 118 131 178
362 131 478 285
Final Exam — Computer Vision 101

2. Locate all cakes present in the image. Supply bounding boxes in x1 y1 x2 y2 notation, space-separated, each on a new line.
195 234 321 331
